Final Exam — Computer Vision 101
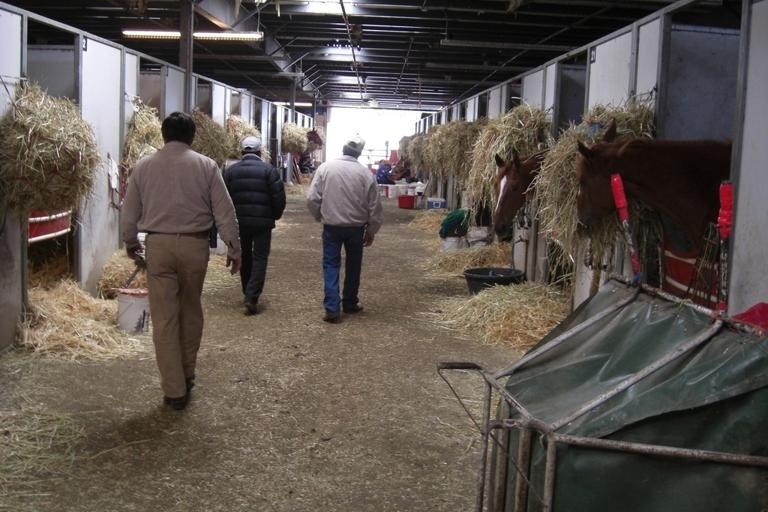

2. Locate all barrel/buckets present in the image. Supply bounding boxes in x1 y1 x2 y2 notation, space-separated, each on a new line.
443 236 459 252
114 288 148 333
376 182 417 199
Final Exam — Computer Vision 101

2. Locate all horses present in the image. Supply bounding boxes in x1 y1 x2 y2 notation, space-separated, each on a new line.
491 146 552 242
574 116 733 238
306 127 323 147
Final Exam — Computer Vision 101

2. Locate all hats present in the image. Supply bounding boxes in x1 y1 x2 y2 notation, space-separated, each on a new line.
345 137 365 153
242 135 261 153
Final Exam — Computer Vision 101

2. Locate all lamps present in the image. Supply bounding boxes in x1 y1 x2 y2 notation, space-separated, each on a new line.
121 0 265 43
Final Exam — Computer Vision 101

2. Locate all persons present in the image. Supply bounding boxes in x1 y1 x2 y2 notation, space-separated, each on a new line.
221 135 287 317
307 134 387 323
118 113 243 411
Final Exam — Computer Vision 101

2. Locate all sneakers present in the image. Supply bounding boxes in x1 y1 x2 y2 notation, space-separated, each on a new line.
346 303 362 313
163 374 196 409
323 315 340 323
246 302 257 314
